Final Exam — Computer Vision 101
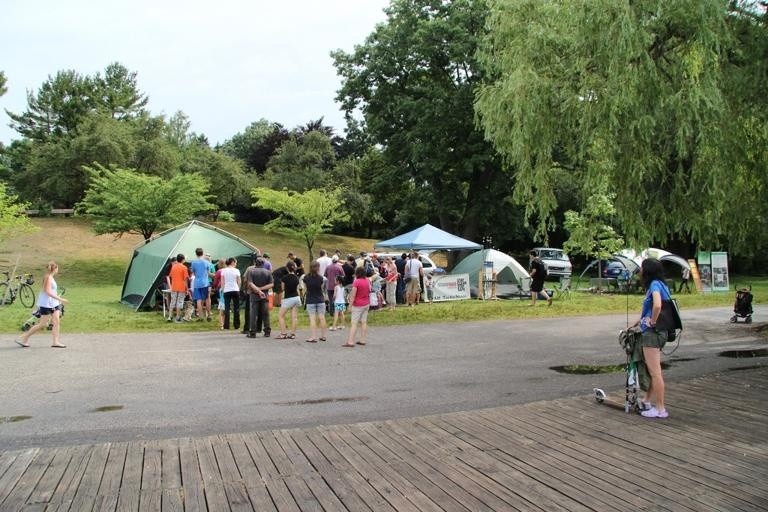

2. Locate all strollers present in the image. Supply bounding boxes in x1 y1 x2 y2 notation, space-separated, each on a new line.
21 287 65 331
729 283 752 324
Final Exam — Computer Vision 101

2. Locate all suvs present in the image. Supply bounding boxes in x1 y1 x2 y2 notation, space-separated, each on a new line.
603 261 624 289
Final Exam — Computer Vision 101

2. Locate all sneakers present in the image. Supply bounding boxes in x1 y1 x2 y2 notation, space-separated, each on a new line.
246 332 271 339
638 397 651 409
167 315 213 323
641 407 669 418
329 325 345 330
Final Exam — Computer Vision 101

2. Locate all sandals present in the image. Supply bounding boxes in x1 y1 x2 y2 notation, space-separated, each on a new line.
275 333 327 343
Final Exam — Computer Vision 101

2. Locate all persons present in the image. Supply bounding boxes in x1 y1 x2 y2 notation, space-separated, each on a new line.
163 248 424 347
15 262 69 348
528 250 552 307
676 267 690 293
634 258 671 418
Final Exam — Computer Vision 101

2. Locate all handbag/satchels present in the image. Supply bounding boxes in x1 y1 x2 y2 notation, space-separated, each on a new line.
368 292 378 307
403 271 411 283
649 297 684 332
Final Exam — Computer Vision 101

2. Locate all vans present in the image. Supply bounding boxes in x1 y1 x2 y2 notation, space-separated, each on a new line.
528 247 572 279
354 251 436 278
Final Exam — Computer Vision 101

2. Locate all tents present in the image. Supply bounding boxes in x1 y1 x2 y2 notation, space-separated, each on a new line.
449 248 534 300
118 220 260 312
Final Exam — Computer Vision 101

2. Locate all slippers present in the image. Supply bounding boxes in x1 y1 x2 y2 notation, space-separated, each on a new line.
51 345 67 349
15 338 31 348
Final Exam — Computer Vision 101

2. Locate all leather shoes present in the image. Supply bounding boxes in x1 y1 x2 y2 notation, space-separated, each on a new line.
342 341 366 348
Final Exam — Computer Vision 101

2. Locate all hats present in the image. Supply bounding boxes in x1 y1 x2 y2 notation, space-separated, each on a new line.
263 252 270 257
286 252 294 257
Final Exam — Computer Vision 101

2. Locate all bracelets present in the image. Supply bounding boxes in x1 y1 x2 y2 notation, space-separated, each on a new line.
649 319 657 327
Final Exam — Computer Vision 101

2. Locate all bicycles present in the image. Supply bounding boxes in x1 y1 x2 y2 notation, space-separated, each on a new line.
0 270 35 309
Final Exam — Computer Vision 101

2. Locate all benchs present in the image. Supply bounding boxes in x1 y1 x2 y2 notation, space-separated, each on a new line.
636 279 675 293
